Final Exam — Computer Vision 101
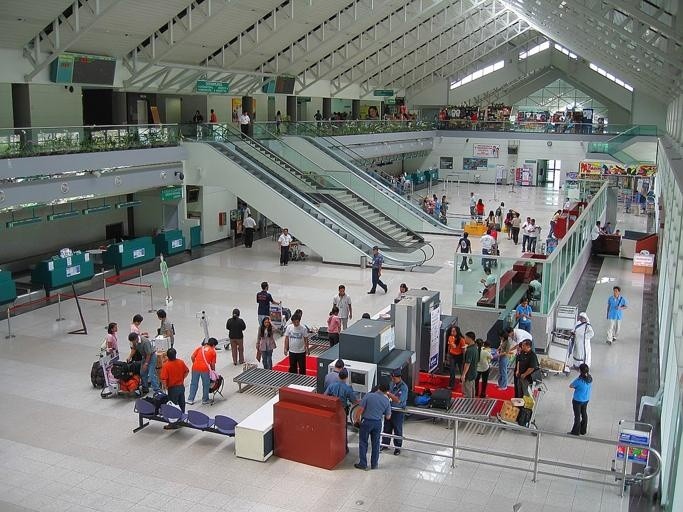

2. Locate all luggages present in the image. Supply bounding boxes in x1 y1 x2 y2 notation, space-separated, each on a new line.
430 388 452 410
487 320 510 349
318 327 329 340
282 308 291 322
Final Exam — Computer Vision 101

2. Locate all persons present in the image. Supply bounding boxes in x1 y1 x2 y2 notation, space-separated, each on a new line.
546 209 560 237
398 284 408 300
273 111 281 133
186 338 218 405
470 192 539 271
418 193 449 222
207 107 218 135
313 109 322 127
238 203 251 218
367 245 387 294
434 109 446 124
570 311 594 369
243 214 256 247
158 253 173 302
366 167 408 191
591 221 621 259
324 359 408 469
193 109 204 139
562 197 569 208
277 228 291 266
455 231 471 270
516 297 533 333
233 103 241 122
327 286 352 346
106 309 190 414
469 113 478 131
446 326 539 399
330 111 351 122
364 104 378 119
529 273 540 300
603 286 628 346
226 282 310 374
506 168 515 192
241 111 251 135
480 268 495 292
567 364 589 436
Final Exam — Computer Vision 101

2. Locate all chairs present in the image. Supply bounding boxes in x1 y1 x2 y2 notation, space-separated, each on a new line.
133 397 235 448
526 284 540 308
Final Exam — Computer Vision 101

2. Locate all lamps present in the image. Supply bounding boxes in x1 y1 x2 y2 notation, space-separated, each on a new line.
173 171 183 180
6 193 144 230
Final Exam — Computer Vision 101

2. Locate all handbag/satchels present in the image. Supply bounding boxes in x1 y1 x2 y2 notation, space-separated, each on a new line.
157 324 175 335
527 369 542 387
414 388 432 408
91 361 223 415
517 406 532 428
242 362 258 372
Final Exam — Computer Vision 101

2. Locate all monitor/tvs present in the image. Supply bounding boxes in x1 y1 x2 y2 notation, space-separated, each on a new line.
351 372 365 385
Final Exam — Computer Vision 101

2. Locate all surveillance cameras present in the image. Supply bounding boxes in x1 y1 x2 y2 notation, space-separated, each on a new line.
179 173 184 180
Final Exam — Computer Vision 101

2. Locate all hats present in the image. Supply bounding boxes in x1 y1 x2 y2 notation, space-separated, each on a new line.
390 370 401 377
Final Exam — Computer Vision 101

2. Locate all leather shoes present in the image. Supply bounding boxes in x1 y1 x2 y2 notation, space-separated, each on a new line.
354 446 400 471
